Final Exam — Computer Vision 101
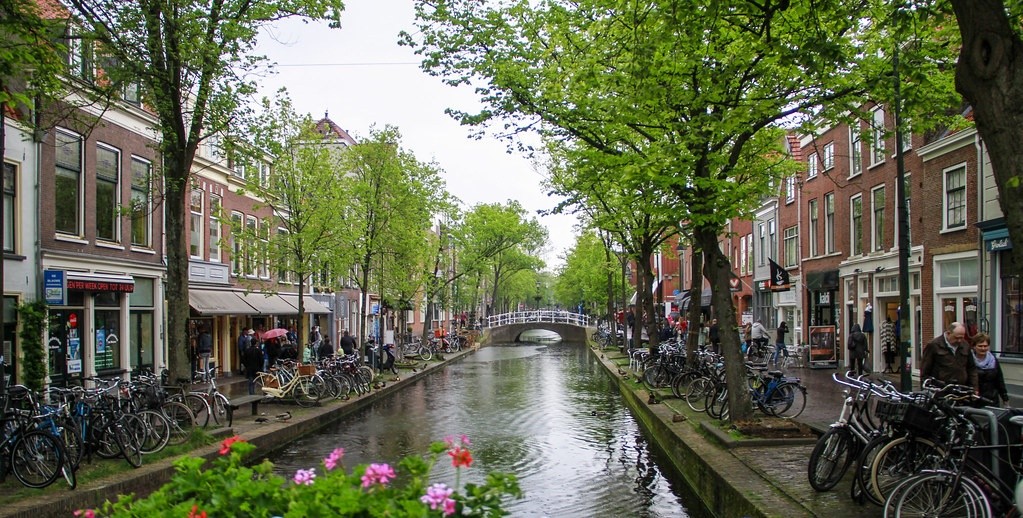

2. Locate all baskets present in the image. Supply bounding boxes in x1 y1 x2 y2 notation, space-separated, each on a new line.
876 400 927 430
299 365 315 374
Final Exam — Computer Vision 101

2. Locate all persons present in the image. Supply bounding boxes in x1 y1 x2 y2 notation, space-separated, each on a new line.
196 325 211 384
847 324 867 376
862 303 872 332
752 319 770 352
662 314 687 340
714 318 720 354
627 309 634 328
242 338 264 395
970 332 1010 408
340 331 356 355
308 326 333 360
239 328 298 375
772 322 789 365
744 322 752 348
618 309 623 323
920 321 979 401
461 310 467 327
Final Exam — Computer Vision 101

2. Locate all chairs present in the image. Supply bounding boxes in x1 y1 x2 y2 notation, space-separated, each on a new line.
629 348 652 371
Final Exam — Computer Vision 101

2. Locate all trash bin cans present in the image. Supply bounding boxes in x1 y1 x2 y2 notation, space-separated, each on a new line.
630 338 643 348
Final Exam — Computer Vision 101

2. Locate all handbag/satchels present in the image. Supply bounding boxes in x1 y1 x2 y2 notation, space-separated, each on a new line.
742 342 747 352
849 334 856 350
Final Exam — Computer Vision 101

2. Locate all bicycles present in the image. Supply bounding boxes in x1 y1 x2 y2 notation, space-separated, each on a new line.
639 336 808 423
250 346 372 409
395 332 461 361
593 324 623 351
0 366 234 492
808 369 1023 518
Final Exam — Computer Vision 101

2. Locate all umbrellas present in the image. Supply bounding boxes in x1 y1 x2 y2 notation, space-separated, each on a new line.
262 329 289 339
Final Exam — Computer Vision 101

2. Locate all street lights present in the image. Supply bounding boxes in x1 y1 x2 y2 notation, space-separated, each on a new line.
536 280 542 309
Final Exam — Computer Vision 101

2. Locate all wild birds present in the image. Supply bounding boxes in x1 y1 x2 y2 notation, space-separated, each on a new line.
276 411 291 421
601 354 604 358
591 345 596 349
673 413 688 422
635 379 641 383
373 383 379 389
419 363 427 370
618 369 626 375
341 393 350 400
413 369 417 373
648 392 661 404
623 376 630 380
396 376 400 381
255 418 268 424
381 381 386 387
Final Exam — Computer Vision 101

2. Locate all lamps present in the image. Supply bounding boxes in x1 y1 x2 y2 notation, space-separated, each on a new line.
944 301 954 312
876 266 884 273
854 269 862 272
966 299 976 311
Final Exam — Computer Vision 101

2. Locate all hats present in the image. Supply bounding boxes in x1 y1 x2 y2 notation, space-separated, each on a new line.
247 329 255 334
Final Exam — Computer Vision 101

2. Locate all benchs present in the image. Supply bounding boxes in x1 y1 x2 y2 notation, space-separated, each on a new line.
224 395 264 420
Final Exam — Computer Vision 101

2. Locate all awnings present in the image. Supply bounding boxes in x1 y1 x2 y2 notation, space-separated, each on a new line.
189 288 332 315
674 291 713 311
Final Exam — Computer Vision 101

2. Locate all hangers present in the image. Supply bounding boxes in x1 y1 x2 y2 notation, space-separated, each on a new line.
883 316 895 324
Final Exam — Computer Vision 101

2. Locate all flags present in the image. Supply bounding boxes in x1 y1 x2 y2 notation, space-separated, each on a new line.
770 260 791 293
730 272 742 292
